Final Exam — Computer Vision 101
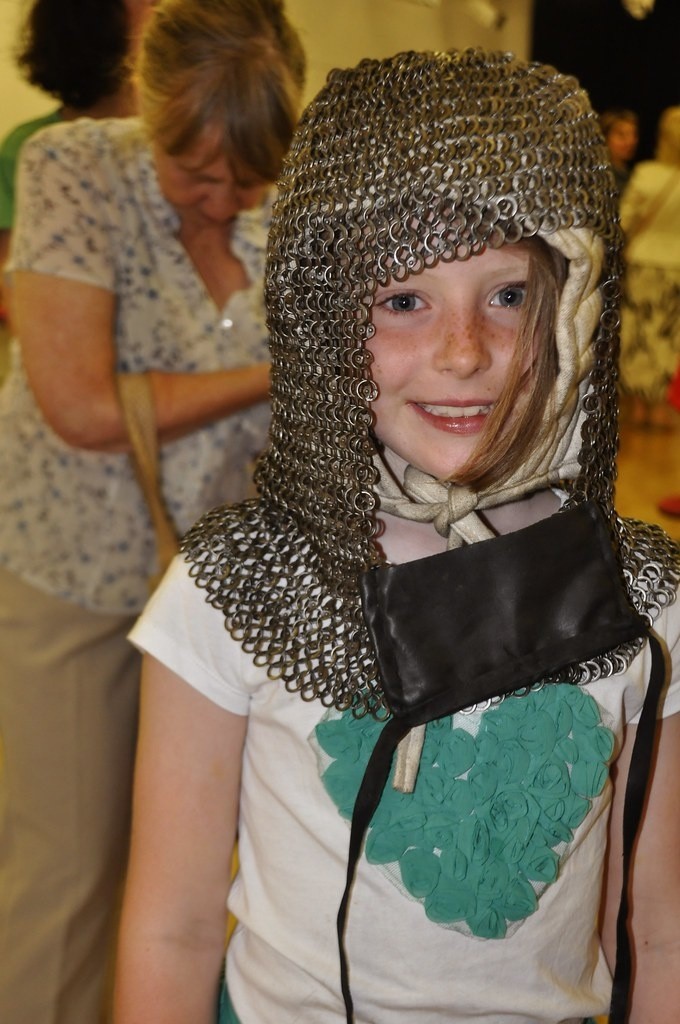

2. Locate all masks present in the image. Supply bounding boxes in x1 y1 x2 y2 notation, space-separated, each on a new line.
337 497 672 1024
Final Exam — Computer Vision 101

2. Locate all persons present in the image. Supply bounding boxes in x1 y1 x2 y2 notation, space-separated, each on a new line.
0 0 679 1023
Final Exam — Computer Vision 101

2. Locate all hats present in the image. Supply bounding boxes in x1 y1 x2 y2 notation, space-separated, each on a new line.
276 111 604 792
